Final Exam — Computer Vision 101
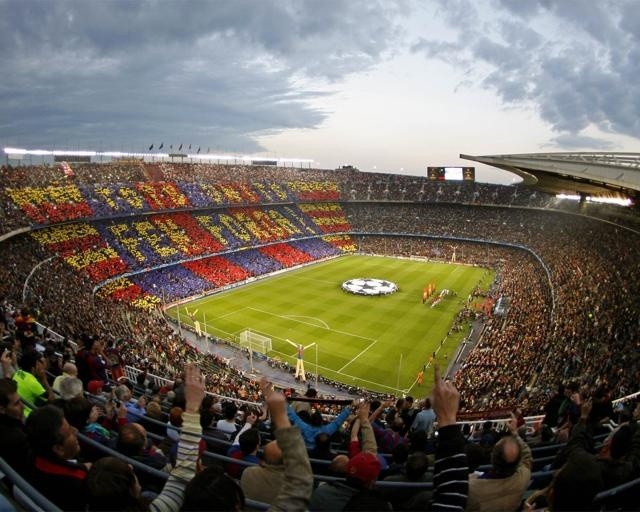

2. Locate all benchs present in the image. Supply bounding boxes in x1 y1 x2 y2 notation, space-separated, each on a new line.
1 371 640 512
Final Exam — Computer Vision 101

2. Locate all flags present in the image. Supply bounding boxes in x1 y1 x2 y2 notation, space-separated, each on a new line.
149 142 210 154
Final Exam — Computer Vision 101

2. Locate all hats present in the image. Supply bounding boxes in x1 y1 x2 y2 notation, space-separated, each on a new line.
348 452 388 480
